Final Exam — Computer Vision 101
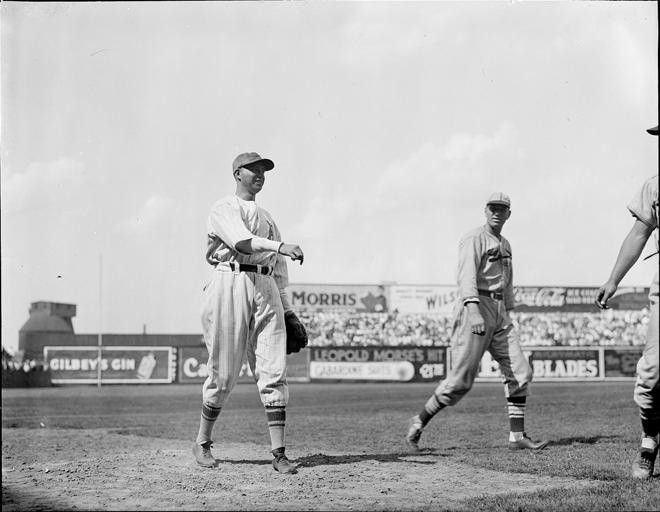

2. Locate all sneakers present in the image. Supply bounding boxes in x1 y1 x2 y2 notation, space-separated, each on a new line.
631 437 658 482
192 439 218 469
272 455 297 475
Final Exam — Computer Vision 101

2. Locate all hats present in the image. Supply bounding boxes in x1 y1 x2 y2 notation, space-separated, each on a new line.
485 191 512 208
232 151 274 176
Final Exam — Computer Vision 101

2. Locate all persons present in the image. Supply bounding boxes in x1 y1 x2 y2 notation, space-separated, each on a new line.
404 190 551 452
192 148 309 476
593 124 659 482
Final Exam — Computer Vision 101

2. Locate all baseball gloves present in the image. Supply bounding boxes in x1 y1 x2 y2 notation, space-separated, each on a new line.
284 311 308 355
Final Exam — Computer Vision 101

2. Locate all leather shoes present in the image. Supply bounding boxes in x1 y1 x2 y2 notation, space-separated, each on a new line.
507 435 552 451
405 414 423 452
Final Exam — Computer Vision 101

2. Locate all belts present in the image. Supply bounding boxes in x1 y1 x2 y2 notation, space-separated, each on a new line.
476 288 504 301
217 262 275 277
